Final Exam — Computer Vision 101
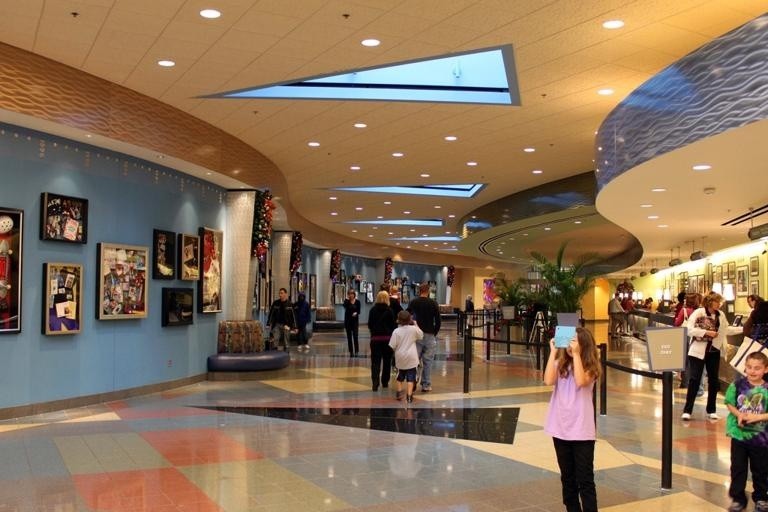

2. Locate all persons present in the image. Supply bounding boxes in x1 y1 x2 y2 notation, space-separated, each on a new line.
466 295 474 329
543 327 603 512
292 291 312 350
724 351 768 511
608 291 653 339
495 305 503 321
673 291 768 421
367 277 438 403
265 288 298 351
344 290 360 358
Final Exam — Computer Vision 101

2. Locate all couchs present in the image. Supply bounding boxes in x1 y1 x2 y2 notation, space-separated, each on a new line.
312 306 345 331
207 320 291 372
438 304 459 319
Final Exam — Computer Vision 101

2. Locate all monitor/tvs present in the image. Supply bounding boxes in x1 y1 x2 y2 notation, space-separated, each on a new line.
722 300 735 314
729 315 743 327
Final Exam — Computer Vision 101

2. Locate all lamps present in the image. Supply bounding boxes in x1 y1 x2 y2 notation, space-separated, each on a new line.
723 284 735 303
713 283 722 294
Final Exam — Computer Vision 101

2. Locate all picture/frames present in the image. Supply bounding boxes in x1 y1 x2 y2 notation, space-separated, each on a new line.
255 269 437 312
750 256 759 276
735 265 749 297
0 192 223 338
704 281 709 294
732 315 743 327
728 261 735 278
716 265 722 281
722 263 728 280
749 280 759 297
697 274 705 308
707 263 713 287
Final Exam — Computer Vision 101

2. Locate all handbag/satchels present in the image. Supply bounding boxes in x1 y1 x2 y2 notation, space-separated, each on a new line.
728 324 768 377
694 316 716 342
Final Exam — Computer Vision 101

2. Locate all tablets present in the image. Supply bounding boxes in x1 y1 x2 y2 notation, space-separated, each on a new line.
554 325 576 349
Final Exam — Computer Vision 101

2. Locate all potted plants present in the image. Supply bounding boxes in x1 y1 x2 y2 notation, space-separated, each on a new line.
484 271 527 342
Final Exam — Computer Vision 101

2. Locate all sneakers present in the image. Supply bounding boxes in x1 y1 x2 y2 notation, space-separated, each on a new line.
421 386 432 392
412 377 419 392
728 502 747 512
681 412 691 419
755 501 768 512
406 395 413 403
706 413 718 419
396 391 405 400
